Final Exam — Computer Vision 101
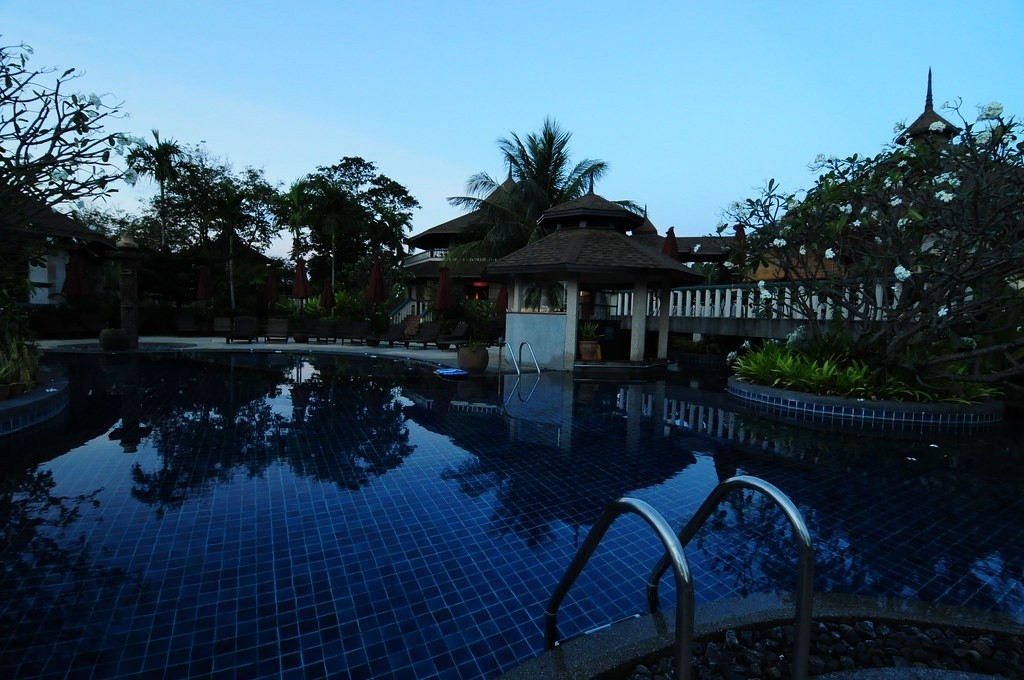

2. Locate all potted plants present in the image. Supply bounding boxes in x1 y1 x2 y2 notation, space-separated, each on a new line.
458 336 489 372
436 323 453 350
366 315 390 346
578 323 599 361
289 316 309 343
0 337 42 401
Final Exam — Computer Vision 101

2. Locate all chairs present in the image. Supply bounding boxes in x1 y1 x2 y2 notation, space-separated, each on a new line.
224 316 258 344
304 314 471 351
176 311 233 338
264 319 288 343
